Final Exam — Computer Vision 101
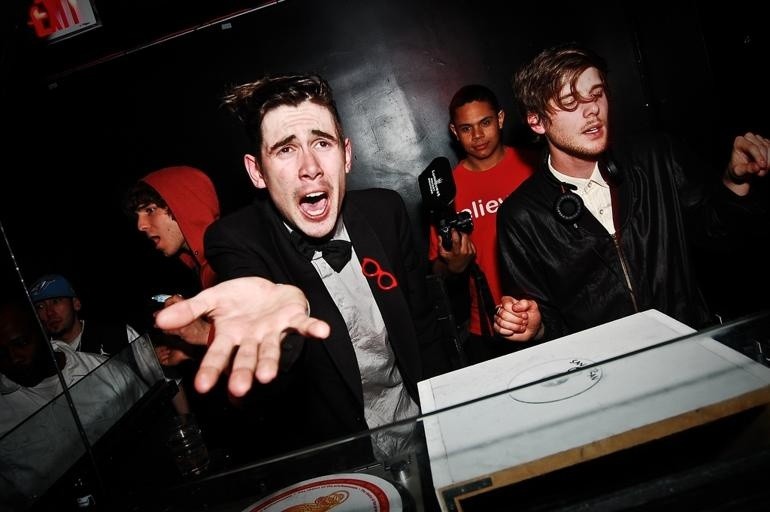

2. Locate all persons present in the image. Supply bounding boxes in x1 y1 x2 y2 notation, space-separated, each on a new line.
25 274 173 388
491 43 769 344
426 83 543 365
121 165 222 370
0 304 153 511
151 75 464 488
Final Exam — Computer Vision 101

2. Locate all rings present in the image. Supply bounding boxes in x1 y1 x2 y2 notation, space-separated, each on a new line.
495 305 503 315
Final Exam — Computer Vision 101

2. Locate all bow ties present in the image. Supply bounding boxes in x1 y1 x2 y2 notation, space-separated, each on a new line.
287 229 353 273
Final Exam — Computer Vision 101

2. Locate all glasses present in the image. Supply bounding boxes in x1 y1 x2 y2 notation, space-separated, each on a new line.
361 257 399 291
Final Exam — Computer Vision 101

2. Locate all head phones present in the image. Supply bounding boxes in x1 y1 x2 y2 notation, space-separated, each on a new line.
541 149 620 223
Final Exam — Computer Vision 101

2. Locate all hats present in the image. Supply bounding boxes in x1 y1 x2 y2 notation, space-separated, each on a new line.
30 276 76 301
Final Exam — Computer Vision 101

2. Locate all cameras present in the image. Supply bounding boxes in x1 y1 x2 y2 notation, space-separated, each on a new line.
417 156 475 251
